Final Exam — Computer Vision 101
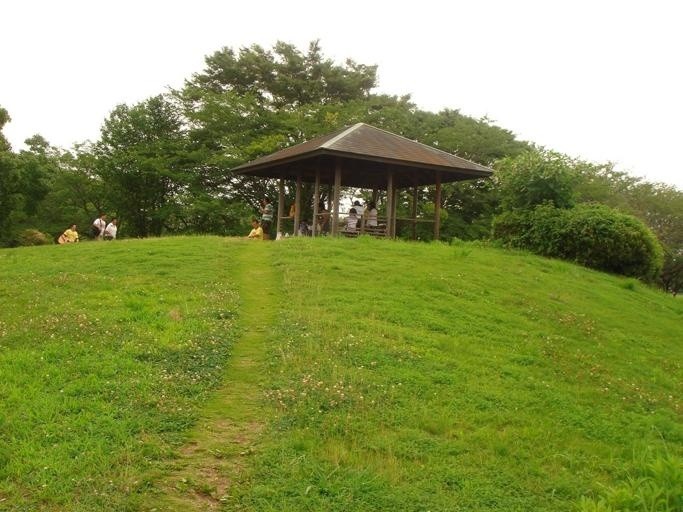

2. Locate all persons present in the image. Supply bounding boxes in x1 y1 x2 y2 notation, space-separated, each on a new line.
61 224 79 243
286 200 378 237
105 217 119 241
93 212 106 241
259 197 274 240
248 217 263 241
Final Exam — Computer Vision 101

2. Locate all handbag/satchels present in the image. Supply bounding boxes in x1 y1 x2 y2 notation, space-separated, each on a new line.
104 231 113 240
91 225 100 236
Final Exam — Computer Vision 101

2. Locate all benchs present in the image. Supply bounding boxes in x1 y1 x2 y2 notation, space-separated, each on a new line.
338 222 386 238
